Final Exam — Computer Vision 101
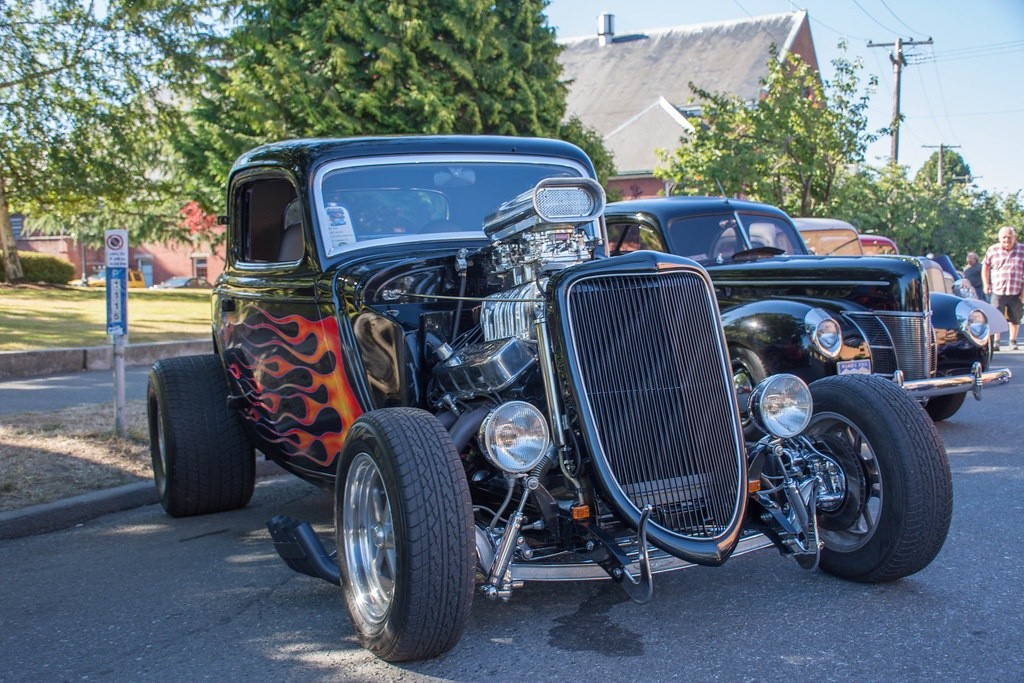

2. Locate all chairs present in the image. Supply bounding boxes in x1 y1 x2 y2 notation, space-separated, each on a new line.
276 222 305 262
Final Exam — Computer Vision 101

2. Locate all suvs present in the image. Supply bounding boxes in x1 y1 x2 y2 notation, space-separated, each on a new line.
68 268 145 288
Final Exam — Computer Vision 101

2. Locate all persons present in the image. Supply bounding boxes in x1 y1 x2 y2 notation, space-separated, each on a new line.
962 252 983 299
980 225 1024 351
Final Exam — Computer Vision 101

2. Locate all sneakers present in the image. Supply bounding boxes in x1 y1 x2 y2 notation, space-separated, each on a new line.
1009 342 1018 350
993 343 999 351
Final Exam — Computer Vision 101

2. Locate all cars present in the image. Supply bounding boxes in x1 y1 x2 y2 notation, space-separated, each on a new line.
147 135 954 663
598 177 1012 441
150 276 213 289
